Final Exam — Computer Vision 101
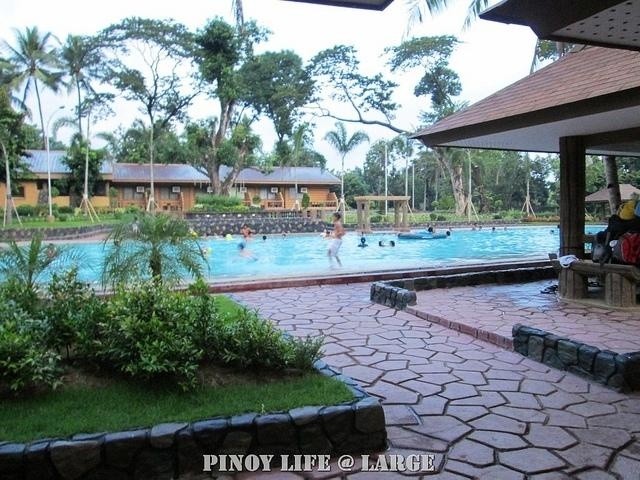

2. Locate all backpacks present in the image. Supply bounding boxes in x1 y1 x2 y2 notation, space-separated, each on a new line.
612 230 640 265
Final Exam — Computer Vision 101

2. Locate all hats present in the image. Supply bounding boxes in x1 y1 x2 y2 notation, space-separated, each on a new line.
629 191 640 200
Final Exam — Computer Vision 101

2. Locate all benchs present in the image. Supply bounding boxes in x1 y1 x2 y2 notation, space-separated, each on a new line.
550 253 640 308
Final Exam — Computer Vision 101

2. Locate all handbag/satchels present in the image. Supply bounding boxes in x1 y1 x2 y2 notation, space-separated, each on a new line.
592 243 611 264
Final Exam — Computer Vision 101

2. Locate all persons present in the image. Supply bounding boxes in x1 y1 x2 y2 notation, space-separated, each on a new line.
446 231 450 239
591 199 639 265
379 241 384 246
491 227 496 232
471 223 478 231
478 225 483 231
325 212 345 268
232 242 259 264
323 231 331 239
318 229 328 237
357 237 367 247
425 225 436 233
200 224 287 242
388 240 395 248
